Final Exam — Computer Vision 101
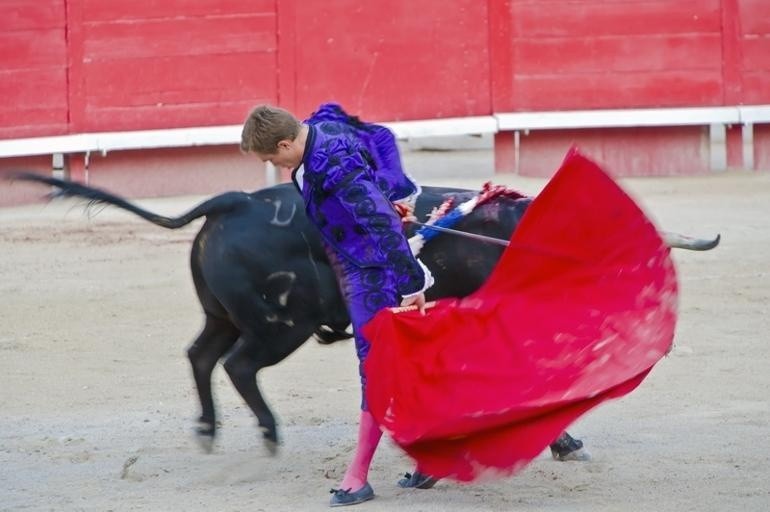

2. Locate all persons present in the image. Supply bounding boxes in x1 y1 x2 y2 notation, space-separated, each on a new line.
239 100 448 509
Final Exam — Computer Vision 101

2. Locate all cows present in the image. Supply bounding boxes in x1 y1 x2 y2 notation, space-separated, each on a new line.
3 170 721 462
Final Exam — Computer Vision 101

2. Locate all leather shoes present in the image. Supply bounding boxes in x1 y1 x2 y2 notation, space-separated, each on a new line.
328 482 375 507
397 469 441 489
